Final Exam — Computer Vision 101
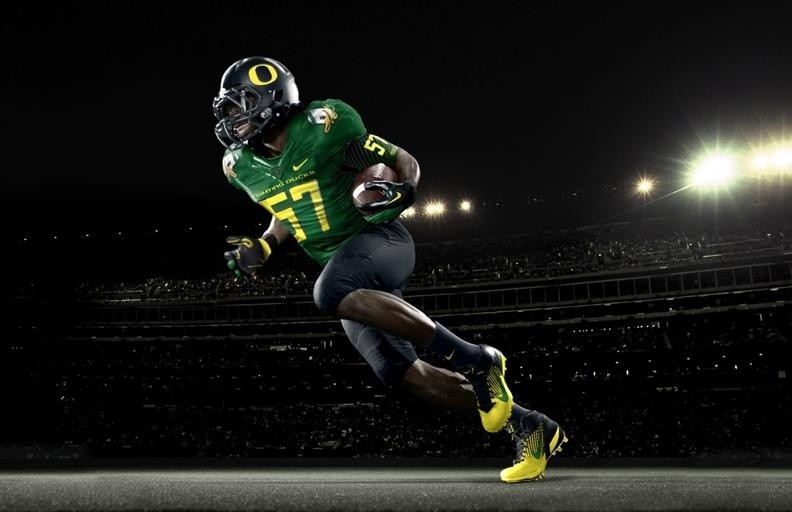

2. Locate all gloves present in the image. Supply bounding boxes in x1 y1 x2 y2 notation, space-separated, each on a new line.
357 180 408 227
223 236 272 287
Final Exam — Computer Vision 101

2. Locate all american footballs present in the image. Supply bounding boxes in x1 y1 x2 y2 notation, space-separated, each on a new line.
352 162 400 217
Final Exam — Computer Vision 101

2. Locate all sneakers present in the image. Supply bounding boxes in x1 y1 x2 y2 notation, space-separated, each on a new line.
500 410 569 482
455 343 514 434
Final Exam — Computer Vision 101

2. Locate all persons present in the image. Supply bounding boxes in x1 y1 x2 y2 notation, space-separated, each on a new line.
1 230 792 463
210 55 570 482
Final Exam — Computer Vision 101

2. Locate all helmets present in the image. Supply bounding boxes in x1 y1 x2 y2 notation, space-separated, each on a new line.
211 57 301 151
